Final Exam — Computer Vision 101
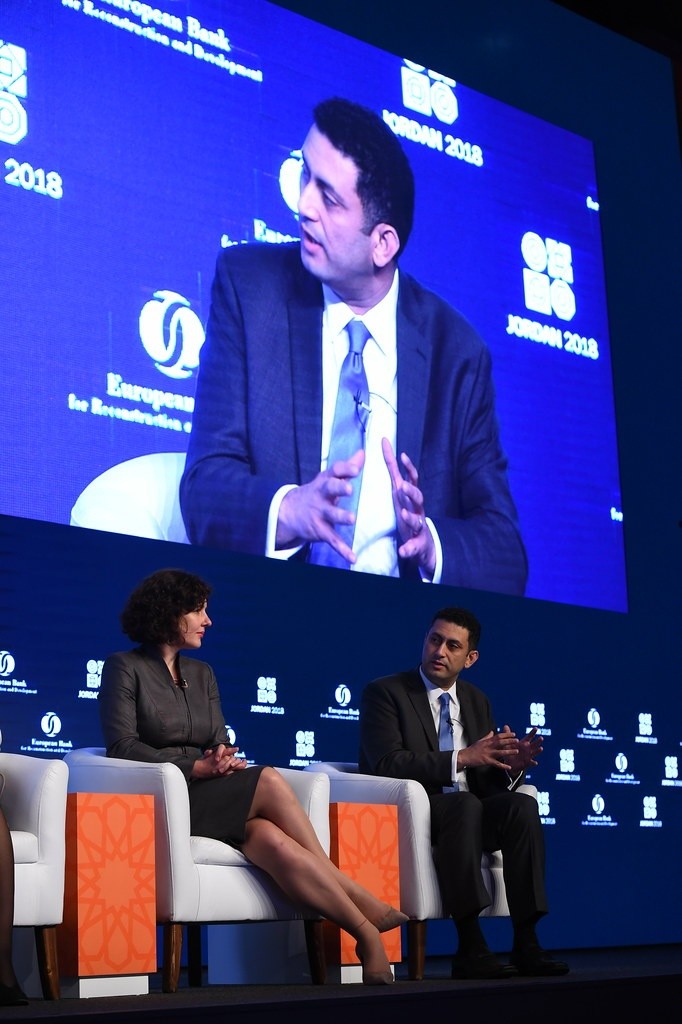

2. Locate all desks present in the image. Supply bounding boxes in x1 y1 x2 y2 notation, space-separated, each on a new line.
308 801 403 985
57 792 158 999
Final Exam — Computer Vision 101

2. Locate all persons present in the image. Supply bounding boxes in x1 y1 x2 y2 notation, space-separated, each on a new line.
98 569 410 984
358 607 569 977
177 96 526 596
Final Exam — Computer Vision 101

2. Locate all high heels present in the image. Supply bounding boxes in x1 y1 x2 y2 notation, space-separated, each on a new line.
373 908 409 933
354 944 393 985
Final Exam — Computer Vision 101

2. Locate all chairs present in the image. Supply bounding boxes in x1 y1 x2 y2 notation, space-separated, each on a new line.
0 745 511 1006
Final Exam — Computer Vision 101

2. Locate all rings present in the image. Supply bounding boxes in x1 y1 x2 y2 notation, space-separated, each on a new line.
531 750 535 755
418 516 423 530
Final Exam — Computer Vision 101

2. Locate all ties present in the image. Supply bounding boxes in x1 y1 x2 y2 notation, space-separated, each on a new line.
437 693 460 794
309 320 371 570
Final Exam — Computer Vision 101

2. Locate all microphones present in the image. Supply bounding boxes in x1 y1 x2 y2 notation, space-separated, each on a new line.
181 678 188 687
446 717 454 726
354 389 361 403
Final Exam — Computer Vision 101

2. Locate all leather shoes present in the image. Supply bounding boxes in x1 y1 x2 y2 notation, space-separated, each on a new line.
509 945 569 976
451 947 503 978
